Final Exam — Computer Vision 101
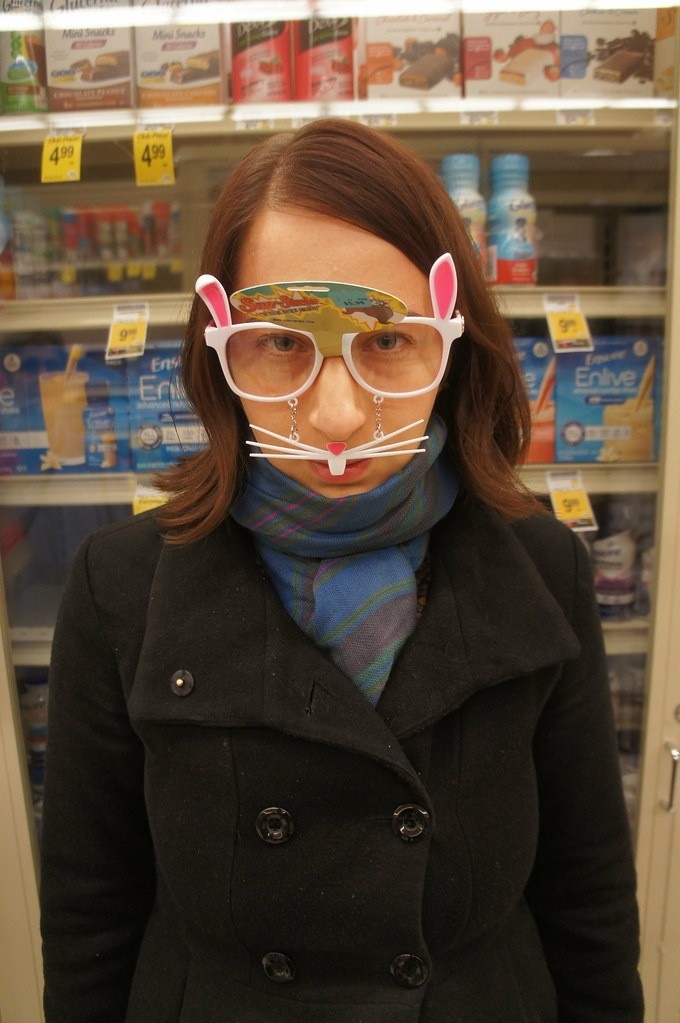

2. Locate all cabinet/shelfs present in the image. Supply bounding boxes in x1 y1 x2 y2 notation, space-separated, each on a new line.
0 108 680 1023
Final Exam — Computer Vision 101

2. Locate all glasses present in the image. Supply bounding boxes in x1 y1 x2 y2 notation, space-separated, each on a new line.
195 253 466 402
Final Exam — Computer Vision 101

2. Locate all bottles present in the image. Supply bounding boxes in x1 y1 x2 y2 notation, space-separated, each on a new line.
82 379 117 468
603 655 646 756
489 152 540 286
19 673 55 792
438 151 489 286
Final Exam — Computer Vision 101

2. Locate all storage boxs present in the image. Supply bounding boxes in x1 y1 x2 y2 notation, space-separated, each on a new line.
0 0 680 477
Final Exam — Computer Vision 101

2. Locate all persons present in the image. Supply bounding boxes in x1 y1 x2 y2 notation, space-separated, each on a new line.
40 121 648 1022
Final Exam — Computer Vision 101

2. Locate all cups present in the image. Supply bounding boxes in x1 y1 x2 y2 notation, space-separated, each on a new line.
602 397 654 461
518 398 556 464
39 371 89 467
